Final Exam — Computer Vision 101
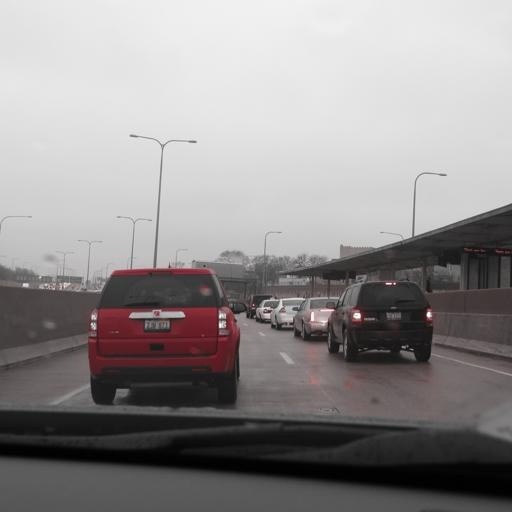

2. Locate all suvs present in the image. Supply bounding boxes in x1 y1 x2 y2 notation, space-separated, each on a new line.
88 261 248 404
246 294 272 319
326 281 434 363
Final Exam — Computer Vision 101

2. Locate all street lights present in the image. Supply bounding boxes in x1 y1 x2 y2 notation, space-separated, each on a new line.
412 172 447 238
380 232 405 241
130 134 197 268
55 251 76 291
77 240 104 289
175 249 188 268
0 215 33 230
117 216 152 270
265 231 282 296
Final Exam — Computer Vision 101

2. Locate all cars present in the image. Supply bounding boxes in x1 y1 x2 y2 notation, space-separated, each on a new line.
255 297 280 323
21 283 67 291
270 298 306 330
292 296 341 341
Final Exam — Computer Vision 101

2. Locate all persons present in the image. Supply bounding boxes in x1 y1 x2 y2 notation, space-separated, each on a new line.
426 277 432 293
